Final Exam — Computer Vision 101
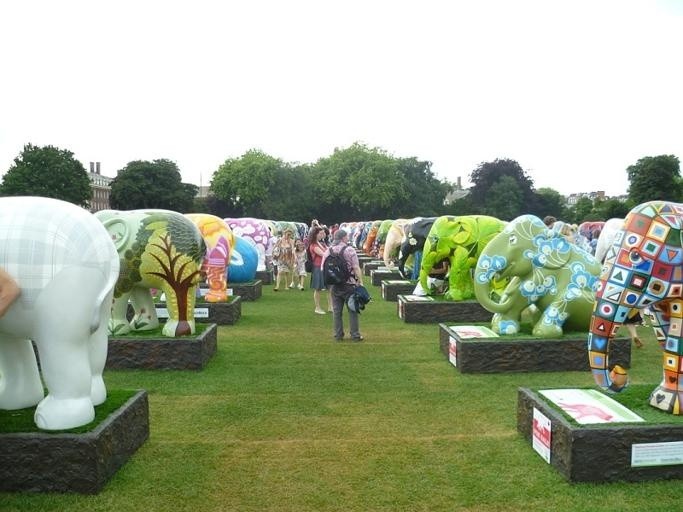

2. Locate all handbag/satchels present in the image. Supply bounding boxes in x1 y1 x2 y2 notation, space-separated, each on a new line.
272 247 281 258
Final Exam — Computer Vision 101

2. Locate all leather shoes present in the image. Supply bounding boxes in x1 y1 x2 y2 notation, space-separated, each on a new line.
315 309 326 314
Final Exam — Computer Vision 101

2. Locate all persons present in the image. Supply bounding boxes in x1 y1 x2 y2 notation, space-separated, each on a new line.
274 218 364 342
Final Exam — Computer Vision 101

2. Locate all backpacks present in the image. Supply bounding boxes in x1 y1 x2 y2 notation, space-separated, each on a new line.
304 243 318 272
323 245 352 285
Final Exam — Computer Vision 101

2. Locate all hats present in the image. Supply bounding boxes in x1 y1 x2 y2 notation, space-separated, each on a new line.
333 230 347 245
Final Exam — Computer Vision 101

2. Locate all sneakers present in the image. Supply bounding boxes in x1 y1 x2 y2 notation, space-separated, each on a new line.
297 283 304 290
289 282 296 288
353 336 363 342
